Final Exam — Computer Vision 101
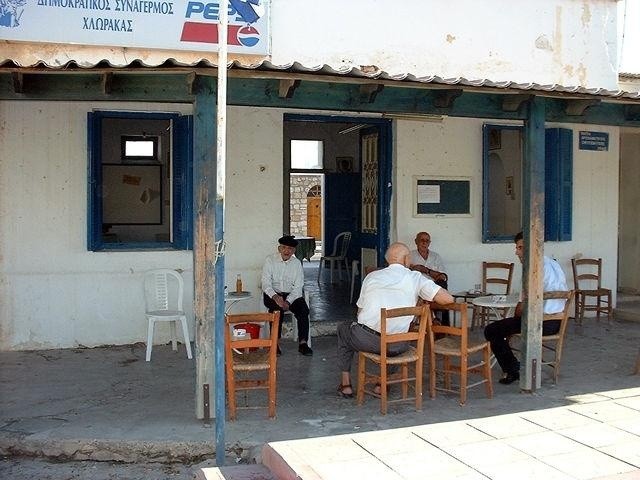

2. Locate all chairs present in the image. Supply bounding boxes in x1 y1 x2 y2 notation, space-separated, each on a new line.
427 300 493 406
571 257 613 327
140 270 193 362
355 303 430 414
260 288 312 349
505 289 574 383
318 232 351 284
470 261 514 331
223 309 281 422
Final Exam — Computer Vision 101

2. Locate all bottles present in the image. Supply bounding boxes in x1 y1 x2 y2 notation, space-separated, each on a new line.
236 274 243 295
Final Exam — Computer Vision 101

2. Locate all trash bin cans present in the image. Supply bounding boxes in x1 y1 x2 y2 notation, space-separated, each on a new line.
233 323 260 351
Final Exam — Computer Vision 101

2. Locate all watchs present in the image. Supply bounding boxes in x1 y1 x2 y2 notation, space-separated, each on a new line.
426 268 431 276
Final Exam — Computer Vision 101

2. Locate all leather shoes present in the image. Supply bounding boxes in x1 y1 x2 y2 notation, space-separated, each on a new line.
299 343 312 356
499 372 519 384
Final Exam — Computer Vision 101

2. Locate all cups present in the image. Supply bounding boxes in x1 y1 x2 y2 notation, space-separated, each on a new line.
469 289 474 294
475 284 481 294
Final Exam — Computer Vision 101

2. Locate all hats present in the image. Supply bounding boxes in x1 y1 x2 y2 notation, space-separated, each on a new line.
279 237 299 247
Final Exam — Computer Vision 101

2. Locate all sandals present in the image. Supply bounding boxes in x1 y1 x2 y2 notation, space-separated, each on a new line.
336 384 352 396
373 383 391 398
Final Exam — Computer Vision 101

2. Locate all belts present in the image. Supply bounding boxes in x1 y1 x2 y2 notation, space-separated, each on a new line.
359 324 381 336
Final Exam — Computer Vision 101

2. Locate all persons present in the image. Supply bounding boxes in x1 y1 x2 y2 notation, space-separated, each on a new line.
337 242 454 399
261 235 313 356
409 232 450 341
484 232 569 384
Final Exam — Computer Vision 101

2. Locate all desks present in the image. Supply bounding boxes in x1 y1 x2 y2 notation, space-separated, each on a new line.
223 290 252 355
293 236 316 265
451 289 491 328
472 293 519 369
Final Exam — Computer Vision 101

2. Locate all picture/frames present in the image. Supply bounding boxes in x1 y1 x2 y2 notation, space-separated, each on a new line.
412 174 474 219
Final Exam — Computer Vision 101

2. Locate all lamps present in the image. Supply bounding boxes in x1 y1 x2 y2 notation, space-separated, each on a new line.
382 112 448 122
338 123 367 135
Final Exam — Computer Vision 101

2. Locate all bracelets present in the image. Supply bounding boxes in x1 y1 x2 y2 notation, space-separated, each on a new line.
285 300 290 305
